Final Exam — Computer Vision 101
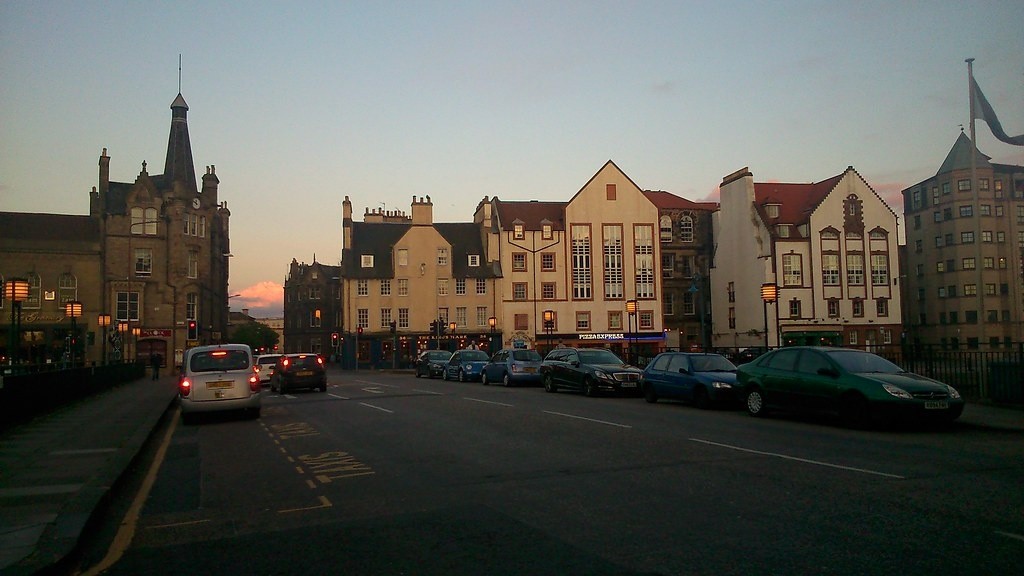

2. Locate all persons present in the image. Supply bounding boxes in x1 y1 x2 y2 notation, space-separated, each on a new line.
468 340 479 350
151 350 163 381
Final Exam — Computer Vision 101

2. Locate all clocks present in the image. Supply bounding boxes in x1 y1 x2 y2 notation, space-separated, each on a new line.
193 198 201 209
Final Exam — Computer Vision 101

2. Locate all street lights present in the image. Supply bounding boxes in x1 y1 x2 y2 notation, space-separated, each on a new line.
63 301 81 370
760 282 794 355
625 299 640 366
98 312 111 367
544 310 554 354
449 321 457 343
688 273 709 349
488 317 496 355
118 321 129 363
5 276 32 378
132 325 142 361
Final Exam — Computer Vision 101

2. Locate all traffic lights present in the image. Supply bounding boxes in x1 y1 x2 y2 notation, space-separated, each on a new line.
187 320 198 341
260 347 265 351
274 345 278 350
439 319 450 338
331 333 339 347
71 336 76 345
390 320 396 333
430 319 439 337
358 327 363 333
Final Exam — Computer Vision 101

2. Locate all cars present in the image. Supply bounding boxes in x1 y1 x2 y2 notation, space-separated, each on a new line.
640 352 742 409
479 349 542 386
178 343 263 417
256 354 287 384
415 349 454 379
735 346 966 432
270 353 328 393
442 349 490 382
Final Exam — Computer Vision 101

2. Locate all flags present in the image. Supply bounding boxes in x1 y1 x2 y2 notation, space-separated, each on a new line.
973 78 1024 146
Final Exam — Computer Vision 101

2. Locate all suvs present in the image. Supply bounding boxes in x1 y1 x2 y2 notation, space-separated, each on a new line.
540 346 644 397
